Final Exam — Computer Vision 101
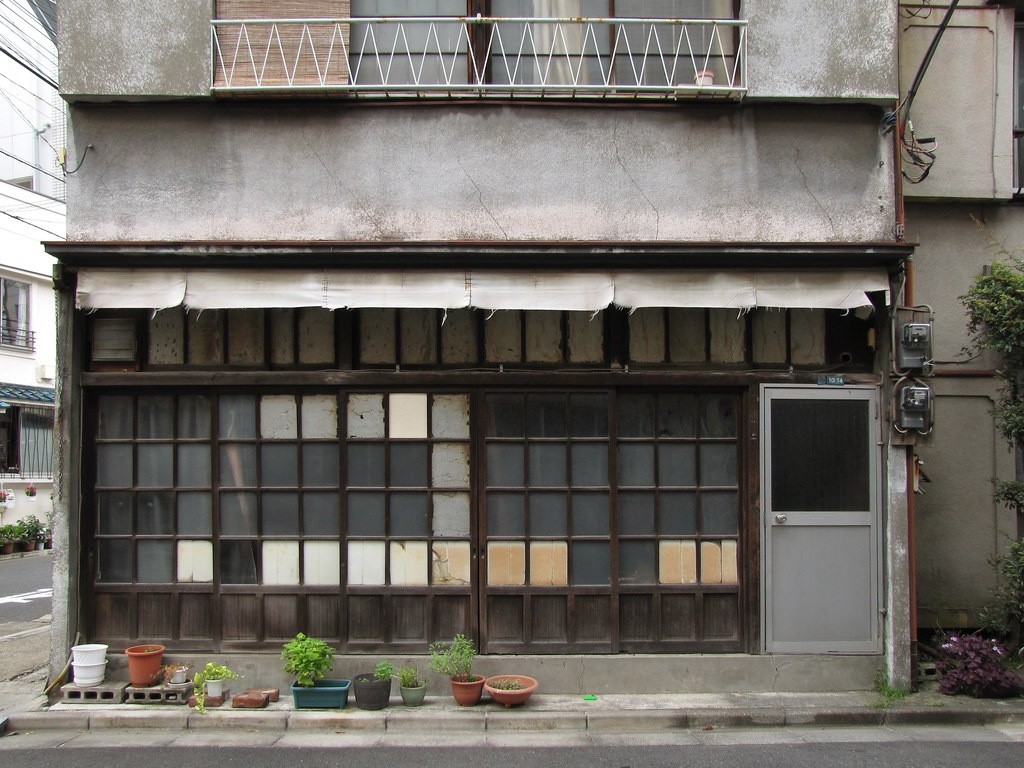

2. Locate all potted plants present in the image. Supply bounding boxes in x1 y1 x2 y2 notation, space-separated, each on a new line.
354 660 393 711
0 493 54 555
694 71 715 85
152 661 194 689
72 643 109 688
193 662 245 714
391 666 429 708
483 674 539 709
126 643 166 689
280 632 351 711
428 633 485 707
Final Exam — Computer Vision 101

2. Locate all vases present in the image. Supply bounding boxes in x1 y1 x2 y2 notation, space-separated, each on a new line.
0 502 9 509
25 495 37 502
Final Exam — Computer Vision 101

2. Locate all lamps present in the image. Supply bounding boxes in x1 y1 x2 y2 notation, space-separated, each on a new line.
59 143 95 174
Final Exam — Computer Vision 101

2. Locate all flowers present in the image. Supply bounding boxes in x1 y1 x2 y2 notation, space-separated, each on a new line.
25 486 38 497
0 488 10 502
